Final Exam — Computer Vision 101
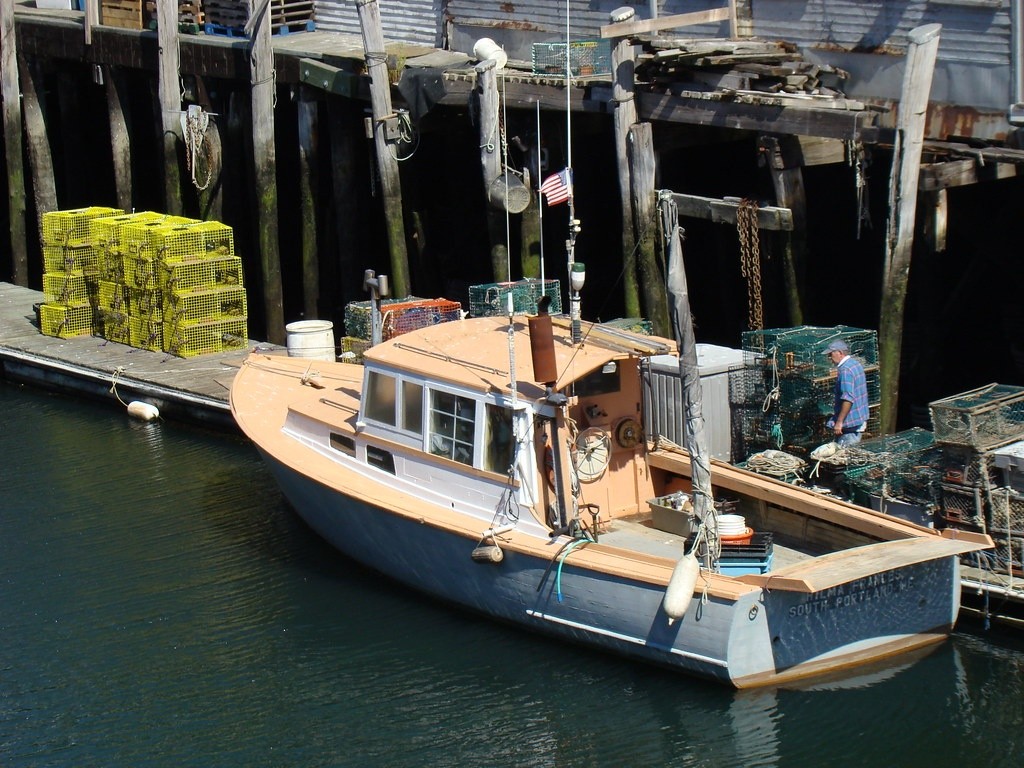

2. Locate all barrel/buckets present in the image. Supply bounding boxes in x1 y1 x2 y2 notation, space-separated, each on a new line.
286 320 337 363
717 515 753 544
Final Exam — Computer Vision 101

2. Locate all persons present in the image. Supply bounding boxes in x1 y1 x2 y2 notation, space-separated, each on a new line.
821 339 870 449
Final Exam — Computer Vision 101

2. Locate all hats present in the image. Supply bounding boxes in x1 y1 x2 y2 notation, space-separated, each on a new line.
820 339 848 354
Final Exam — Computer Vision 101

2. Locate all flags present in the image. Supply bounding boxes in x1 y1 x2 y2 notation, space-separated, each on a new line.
538 168 574 206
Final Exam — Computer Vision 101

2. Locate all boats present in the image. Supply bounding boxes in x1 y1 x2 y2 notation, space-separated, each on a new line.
228 1 1024 692
